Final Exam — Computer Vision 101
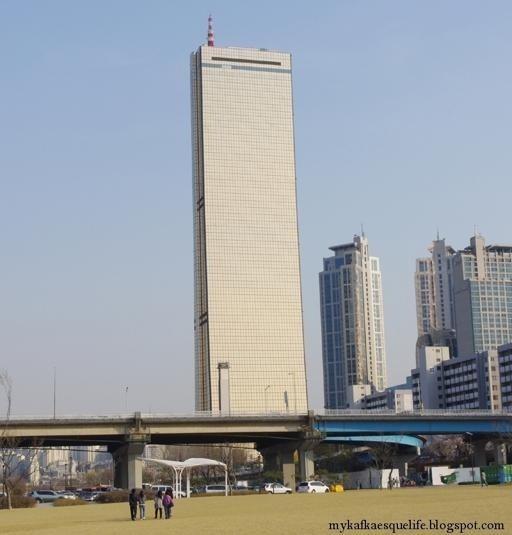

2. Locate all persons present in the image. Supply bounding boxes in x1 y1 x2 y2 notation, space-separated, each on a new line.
392 477 399 487
153 490 163 519
163 488 174 519
129 489 139 520
481 469 488 487
356 479 360 491
138 490 145 520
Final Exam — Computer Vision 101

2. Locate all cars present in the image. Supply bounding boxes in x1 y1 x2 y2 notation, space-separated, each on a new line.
298 481 329 495
28 481 261 503
259 482 293 495
